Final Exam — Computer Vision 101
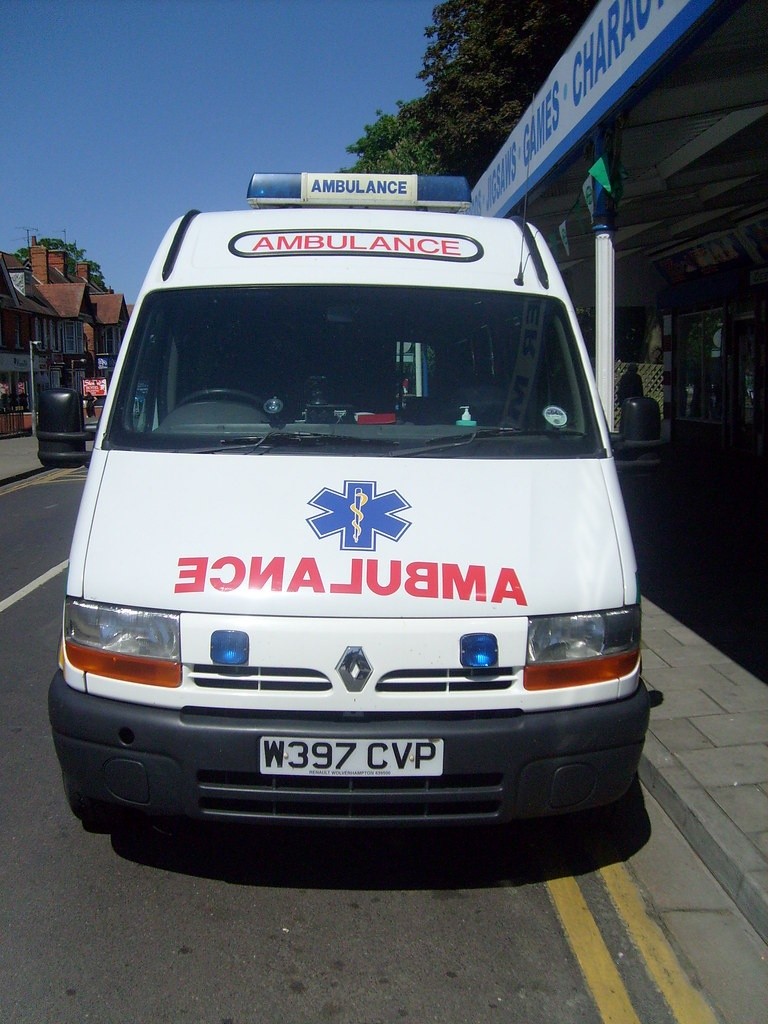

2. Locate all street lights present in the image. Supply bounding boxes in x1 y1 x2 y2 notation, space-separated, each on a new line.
30 340 42 437
73 359 86 389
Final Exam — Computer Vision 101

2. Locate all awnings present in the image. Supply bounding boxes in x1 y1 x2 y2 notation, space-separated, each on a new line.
99 358 116 370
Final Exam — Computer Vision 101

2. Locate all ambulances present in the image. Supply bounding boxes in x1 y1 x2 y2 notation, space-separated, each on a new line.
38 171 662 839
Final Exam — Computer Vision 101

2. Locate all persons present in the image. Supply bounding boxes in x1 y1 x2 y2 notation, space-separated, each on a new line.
83 392 98 418
617 364 643 406
0 393 29 410
